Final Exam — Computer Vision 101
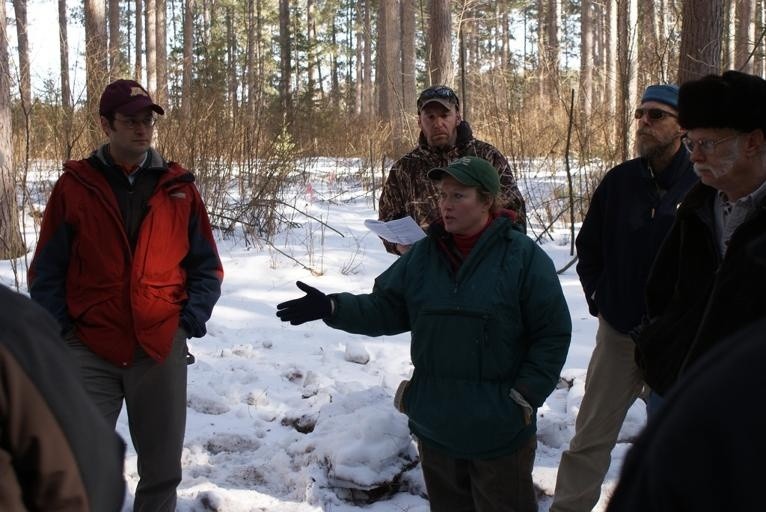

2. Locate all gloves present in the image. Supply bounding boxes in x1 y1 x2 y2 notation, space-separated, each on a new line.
277 281 332 325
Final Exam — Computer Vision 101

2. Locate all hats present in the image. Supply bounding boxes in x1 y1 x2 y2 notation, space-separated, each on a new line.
428 156 499 195
417 86 460 115
641 83 679 109
678 70 766 131
100 79 164 117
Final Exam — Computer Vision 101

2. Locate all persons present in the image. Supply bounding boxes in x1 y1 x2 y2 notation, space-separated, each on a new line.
537 84 703 512
378 86 525 255
1 278 125 512
606 310 766 512
25 78 224 512
275 154 572 512
636 71 766 421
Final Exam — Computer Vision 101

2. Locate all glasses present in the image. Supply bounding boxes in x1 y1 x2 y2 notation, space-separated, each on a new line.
635 109 678 121
114 117 158 128
681 135 738 153
